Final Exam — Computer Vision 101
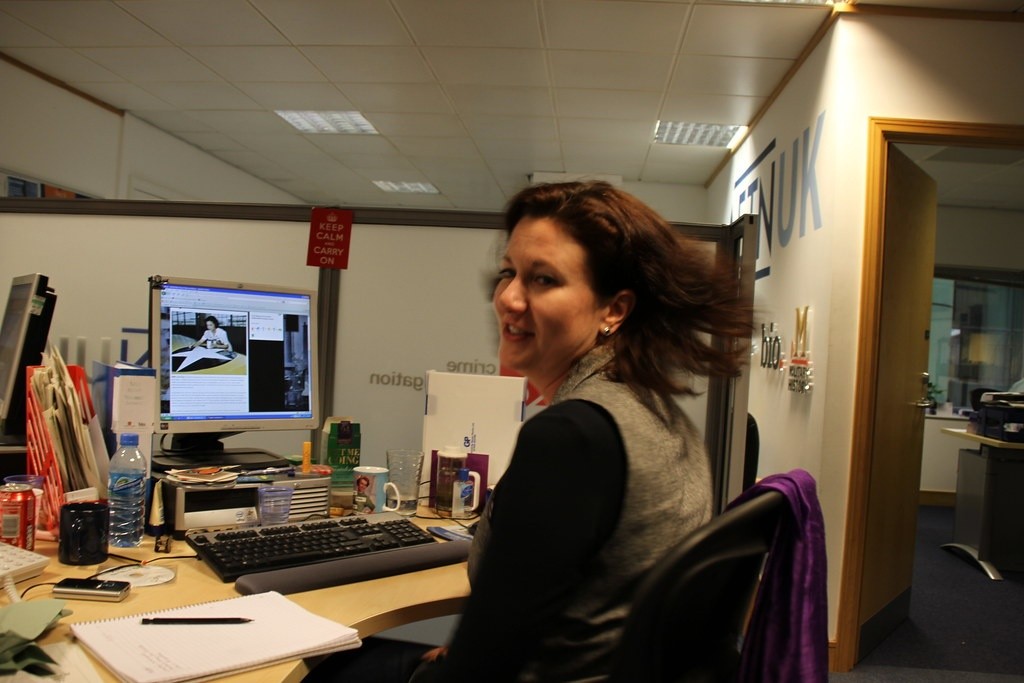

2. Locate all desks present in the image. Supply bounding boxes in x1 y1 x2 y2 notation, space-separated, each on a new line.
939 426 1024 581
1 503 481 683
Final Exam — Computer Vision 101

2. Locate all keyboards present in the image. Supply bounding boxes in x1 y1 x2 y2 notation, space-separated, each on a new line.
185 511 438 583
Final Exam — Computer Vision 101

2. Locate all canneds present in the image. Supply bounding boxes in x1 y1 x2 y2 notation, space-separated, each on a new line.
0 484 36 553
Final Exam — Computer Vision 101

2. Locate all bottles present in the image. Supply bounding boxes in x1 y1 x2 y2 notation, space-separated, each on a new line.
108 432 147 547
453 468 474 519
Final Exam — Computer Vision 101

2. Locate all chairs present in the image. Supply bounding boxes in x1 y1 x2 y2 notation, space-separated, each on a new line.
613 468 815 683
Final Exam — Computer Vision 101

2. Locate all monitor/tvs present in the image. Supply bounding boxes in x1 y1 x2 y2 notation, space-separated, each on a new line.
0 273 58 446
148 274 319 474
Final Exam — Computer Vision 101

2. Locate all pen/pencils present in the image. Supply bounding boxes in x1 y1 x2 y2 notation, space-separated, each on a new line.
141 618 255 624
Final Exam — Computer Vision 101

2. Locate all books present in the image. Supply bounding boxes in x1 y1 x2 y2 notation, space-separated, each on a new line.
72 590 362 683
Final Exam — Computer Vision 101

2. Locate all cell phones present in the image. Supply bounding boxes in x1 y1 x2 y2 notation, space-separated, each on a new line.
53 577 132 602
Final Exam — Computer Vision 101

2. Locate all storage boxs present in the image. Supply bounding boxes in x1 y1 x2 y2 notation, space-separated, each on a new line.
320 415 362 485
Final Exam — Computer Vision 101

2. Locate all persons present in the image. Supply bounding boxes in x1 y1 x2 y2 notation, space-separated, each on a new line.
411 180 752 683
353 477 376 515
191 316 233 352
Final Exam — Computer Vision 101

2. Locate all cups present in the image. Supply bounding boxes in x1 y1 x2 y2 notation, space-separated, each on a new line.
258 486 293 526
5 475 44 539
433 445 481 515
58 502 109 565
386 449 425 515
353 466 400 516
